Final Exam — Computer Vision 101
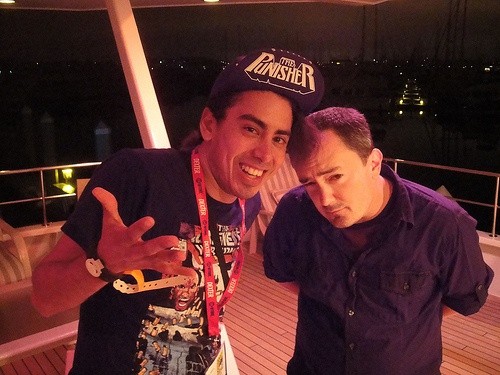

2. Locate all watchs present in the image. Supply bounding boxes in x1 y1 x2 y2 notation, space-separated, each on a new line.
85 249 116 283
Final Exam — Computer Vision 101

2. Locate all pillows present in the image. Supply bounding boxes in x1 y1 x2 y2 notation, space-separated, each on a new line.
271 189 287 204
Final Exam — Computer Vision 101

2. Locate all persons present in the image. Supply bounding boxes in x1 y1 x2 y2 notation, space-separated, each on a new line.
264 108 494 375
30 48 327 375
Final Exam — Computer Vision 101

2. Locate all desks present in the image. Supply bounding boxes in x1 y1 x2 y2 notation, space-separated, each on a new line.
0 278 80 375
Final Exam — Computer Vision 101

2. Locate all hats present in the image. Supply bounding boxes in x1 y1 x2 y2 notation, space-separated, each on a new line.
207 48 325 117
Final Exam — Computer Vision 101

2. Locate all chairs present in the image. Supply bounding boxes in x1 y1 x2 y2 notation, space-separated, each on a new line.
249 153 303 255
0 220 32 286
